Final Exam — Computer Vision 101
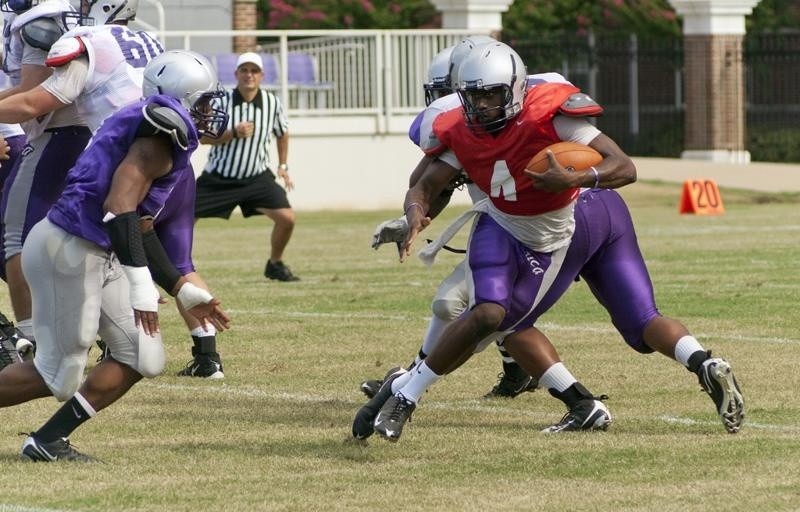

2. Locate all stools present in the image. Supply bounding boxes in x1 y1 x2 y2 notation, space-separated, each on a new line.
261 55 282 97
216 52 239 87
289 54 332 107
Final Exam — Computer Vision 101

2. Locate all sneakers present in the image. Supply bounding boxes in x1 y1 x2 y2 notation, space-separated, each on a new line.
485 373 538 397
19 433 98 464
265 260 297 282
360 379 383 399
698 357 745 434
351 370 409 441
10 329 34 361
373 391 416 442
541 398 612 433
178 352 224 378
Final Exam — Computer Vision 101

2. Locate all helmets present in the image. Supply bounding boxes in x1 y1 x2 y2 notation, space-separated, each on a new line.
455 41 528 135
423 46 453 106
142 50 229 138
450 37 496 92
62 1 138 31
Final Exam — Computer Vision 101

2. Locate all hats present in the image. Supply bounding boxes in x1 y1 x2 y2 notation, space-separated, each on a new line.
234 51 263 72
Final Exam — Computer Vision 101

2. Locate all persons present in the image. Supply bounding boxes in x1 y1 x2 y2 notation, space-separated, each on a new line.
361 43 544 399
408 36 746 437
1 0 120 365
0 49 228 473
0 43 29 368
196 51 301 284
0 0 227 380
351 44 639 443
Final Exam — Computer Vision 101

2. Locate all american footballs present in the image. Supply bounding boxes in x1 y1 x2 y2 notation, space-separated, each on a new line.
526 143 602 184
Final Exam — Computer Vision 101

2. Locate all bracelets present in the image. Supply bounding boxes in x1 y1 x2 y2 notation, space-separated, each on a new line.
405 203 426 217
590 166 600 190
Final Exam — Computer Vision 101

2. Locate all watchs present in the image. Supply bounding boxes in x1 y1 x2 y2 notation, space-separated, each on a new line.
277 163 288 171
